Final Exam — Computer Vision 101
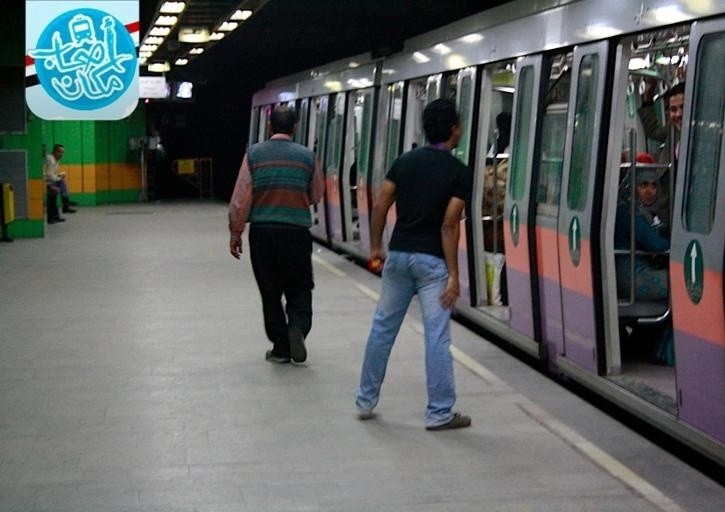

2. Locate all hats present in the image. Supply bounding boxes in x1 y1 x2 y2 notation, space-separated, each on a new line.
624 151 661 199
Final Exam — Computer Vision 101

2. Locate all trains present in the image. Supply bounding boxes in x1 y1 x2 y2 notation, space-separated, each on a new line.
248 0 725 464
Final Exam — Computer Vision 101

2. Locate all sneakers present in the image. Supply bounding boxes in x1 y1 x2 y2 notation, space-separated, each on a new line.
48 200 78 223
287 324 307 365
264 341 288 363
424 413 472 431
354 403 372 419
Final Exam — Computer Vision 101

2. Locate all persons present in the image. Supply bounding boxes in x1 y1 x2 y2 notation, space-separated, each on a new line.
46 142 83 213
46 186 66 224
224 104 325 368
638 71 690 164
353 96 474 431
487 109 514 156
615 144 673 369
